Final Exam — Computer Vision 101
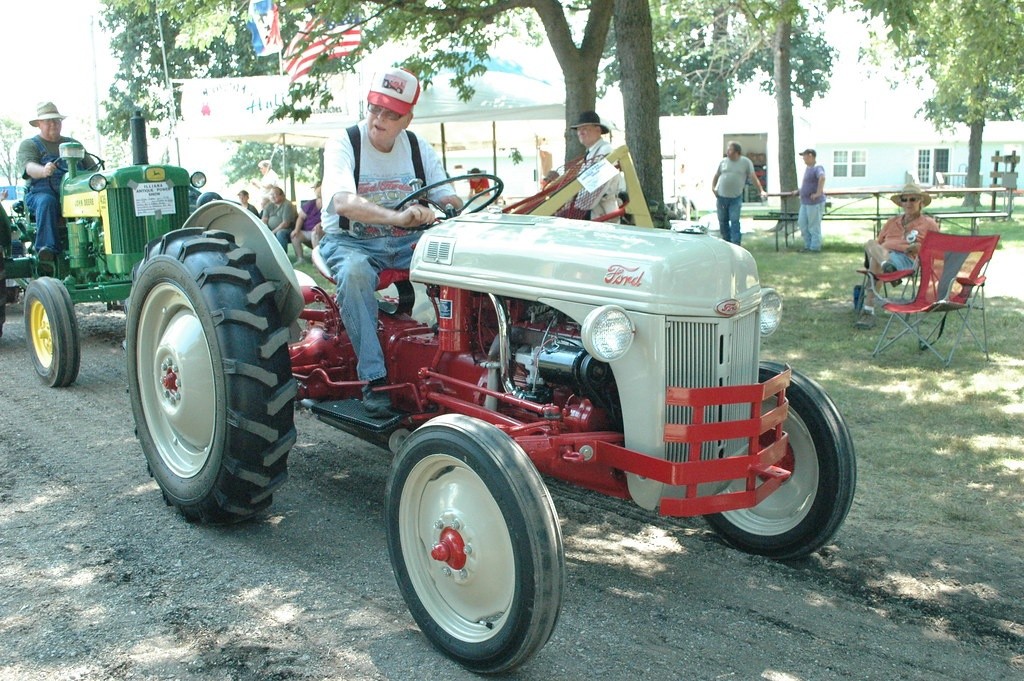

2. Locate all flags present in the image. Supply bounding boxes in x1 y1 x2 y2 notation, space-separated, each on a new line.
246 0 284 57
282 0 362 86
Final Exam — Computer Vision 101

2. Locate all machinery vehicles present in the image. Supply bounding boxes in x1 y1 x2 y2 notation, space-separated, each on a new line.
0 142 212 389
122 173 858 675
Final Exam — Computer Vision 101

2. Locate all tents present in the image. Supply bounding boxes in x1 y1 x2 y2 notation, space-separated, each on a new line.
171 69 603 271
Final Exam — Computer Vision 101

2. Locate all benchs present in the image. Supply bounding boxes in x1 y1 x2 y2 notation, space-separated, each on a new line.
754 209 1009 252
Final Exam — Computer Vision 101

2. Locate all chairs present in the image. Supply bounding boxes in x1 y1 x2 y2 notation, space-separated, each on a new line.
851 217 941 325
871 230 1001 368
301 200 312 250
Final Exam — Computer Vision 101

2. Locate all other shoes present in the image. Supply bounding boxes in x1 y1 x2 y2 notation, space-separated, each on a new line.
856 310 876 329
38 246 55 271
882 262 902 287
798 249 819 253
293 257 305 269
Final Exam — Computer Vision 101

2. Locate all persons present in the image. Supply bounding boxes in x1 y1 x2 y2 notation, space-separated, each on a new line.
467 168 491 200
237 159 324 267
791 148 826 255
712 141 769 247
17 101 100 272
569 111 621 225
318 67 464 417
855 183 940 330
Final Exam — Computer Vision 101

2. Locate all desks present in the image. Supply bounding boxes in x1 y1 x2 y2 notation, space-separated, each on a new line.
766 187 1007 248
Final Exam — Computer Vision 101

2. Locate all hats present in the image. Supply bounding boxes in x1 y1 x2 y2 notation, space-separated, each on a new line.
570 112 610 135
367 67 420 114
799 149 816 157
890 183 932 208
28 102 68 128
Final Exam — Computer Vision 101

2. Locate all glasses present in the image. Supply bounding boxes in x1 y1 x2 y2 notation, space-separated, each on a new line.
901 198 919 202
368 104 403 120
42 119 60 124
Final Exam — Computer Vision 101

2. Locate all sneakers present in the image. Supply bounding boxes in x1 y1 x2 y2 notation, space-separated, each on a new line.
362 380 394 418
